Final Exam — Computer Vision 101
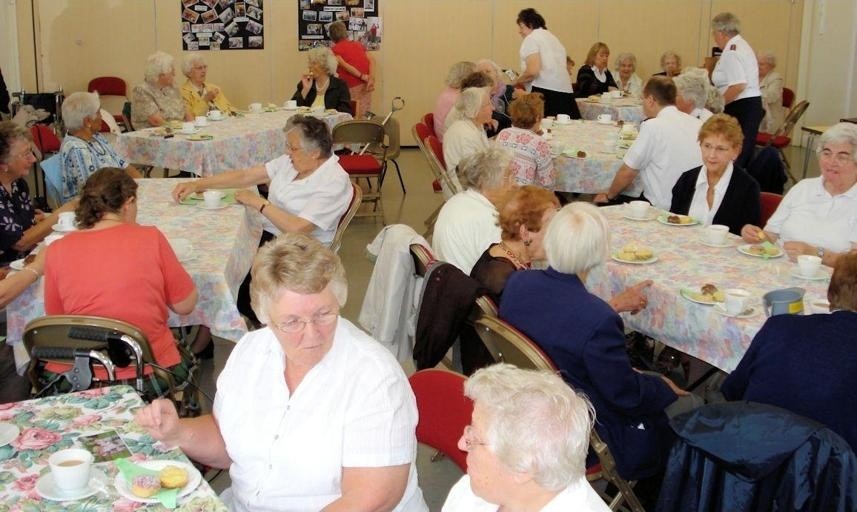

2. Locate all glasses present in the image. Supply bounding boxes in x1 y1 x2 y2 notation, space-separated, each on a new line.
267 307 338 334
464 426 488 450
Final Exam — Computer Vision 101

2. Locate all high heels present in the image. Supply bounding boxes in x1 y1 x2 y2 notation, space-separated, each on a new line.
195 338 213 360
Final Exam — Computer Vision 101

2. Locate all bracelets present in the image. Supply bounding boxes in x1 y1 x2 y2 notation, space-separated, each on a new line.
22 265 42 280
359 73 363 79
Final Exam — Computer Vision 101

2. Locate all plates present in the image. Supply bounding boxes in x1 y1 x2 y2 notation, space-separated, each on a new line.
190 191 225 200
610 96 624 99
597 122 613 125
0 423 20 447
199 202 228 209
610 207 829 318
566 130 638 160
114 460 201 503
162 106 330 141
51 224 80 232
9 258 26 271
35 467 107 501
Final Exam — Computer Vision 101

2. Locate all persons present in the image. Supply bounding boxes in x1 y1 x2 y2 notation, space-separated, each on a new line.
329 20 376 120
290 46 352 121
60 89 144 201
180 54 237 119
131 233 431 512
430 3 780 204
0 122 81 267
173 114 354 361
0 246 46 406
441 362 617 512
35 167 202 418
434 116 857 487
130 52 185 131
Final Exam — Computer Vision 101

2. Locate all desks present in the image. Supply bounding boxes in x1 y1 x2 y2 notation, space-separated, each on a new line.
800 126 832 179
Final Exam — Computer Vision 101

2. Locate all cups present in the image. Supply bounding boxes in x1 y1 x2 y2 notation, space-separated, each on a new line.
166 238 193 259
182 100 325 134
612 91 624 96
597 114 611 122
49 449 95 490
203 191 225 207
540 114 570 154
58 212 80 228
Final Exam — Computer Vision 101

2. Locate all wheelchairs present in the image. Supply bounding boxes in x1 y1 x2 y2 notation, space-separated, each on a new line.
10 88 68 140
31 326 226 485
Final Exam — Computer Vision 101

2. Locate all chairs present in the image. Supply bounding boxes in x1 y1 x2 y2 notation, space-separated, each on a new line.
754 87 810 230
20 315 206 418
28 124 61 212
88 77 134 133
332 112 459 251
659 403 856 511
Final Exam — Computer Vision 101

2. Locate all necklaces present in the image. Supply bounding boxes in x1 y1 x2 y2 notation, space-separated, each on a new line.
315 79 329 93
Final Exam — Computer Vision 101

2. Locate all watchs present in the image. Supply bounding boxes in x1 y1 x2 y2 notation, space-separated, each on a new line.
258 200 271 213
